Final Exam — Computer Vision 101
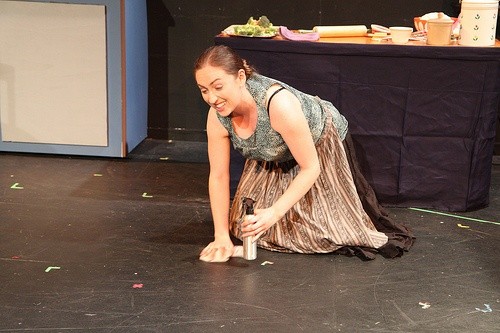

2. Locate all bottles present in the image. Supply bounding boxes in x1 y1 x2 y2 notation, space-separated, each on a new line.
240 197 257 260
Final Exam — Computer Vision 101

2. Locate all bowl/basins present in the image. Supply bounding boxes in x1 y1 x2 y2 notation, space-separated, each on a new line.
413 16 460 36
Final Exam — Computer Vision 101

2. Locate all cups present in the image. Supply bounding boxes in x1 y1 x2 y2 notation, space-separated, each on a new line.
426 12 454 45
389 27 413 43
457 1 499 46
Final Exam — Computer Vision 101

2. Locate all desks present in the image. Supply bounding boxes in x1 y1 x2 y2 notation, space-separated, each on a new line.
215 30 500 213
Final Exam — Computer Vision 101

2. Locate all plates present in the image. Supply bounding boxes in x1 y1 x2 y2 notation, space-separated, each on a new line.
224 23 279 38
